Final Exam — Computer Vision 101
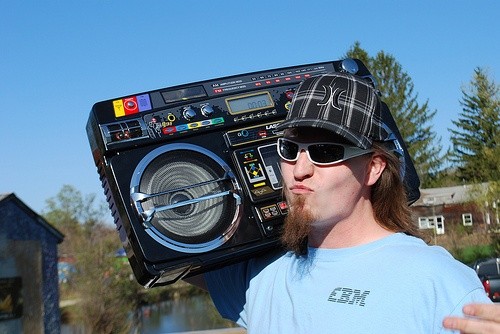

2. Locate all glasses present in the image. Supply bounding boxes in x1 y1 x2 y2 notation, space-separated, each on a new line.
277 138 375 165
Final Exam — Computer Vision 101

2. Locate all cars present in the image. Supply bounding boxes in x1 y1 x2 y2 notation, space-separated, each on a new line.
473 257 500 302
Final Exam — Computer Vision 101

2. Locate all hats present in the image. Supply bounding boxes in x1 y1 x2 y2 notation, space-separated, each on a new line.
271 70 384 150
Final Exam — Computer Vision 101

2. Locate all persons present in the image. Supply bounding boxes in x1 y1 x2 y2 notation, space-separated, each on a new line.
181 72 500 334
